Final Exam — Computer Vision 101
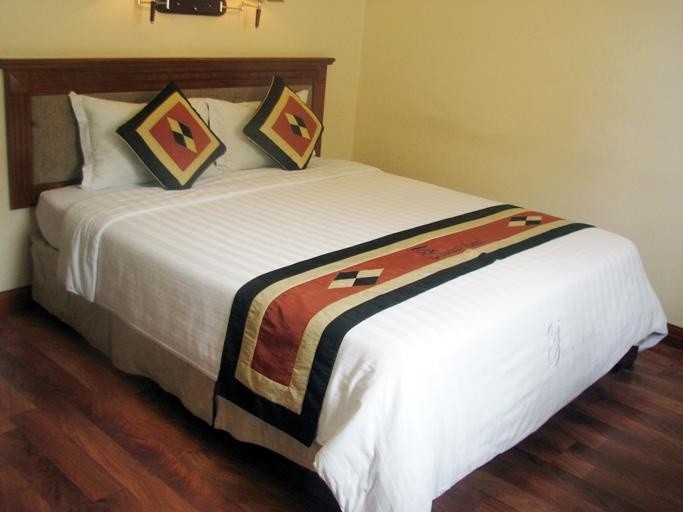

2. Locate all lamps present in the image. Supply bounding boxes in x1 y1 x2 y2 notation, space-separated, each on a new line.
137 0 264 28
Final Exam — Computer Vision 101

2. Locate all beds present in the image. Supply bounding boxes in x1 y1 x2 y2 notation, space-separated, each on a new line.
1 56 669 512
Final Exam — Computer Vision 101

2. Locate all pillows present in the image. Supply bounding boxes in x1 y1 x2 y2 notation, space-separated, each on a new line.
244 75 325 173
69 91 216 189
207 89 309 172
116 82 227 190
